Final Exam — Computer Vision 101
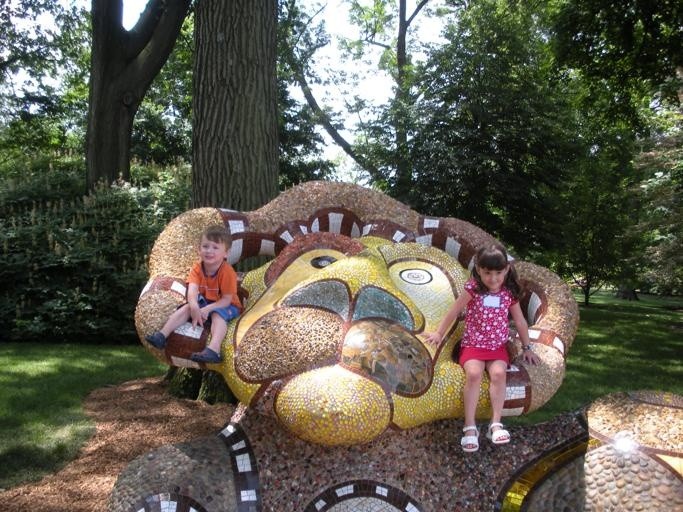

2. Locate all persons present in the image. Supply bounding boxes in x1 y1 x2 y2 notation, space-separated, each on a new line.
420 240 542 453
143 224 245 364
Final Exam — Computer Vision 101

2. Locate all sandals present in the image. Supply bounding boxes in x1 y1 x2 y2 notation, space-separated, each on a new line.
485 423 511 447
192 346 223 366
459 424 481 453
145 330 168 351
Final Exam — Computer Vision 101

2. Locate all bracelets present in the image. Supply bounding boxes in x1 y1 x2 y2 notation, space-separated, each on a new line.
519 342 535 350
